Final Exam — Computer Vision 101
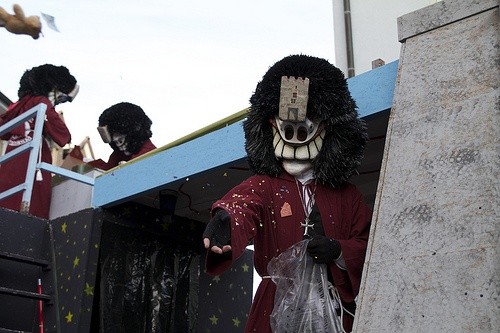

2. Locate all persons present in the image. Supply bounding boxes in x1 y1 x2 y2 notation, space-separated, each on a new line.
0 64 80 219
87 102 157 171
203 54 373 333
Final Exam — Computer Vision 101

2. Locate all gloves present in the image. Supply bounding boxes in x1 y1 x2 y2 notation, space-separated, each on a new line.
306 227 341 265
